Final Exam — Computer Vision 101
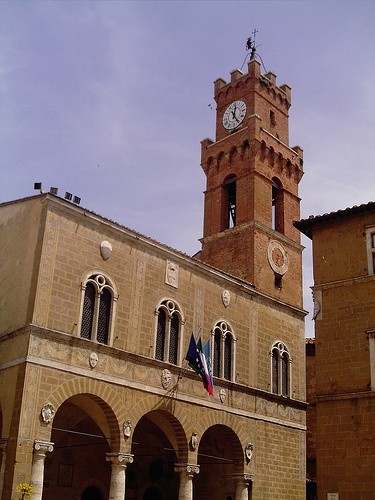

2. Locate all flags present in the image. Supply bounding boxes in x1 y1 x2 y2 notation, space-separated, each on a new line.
183 332 214 397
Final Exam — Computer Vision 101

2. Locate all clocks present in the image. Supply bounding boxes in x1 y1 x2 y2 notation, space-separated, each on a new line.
222 100 247 130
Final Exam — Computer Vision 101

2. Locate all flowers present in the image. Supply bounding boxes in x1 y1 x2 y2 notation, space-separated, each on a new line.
16 483 36 500
